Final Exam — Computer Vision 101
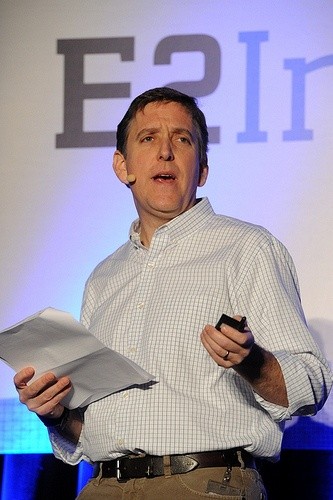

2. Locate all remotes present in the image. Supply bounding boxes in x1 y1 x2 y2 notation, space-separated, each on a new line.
215 314 246 332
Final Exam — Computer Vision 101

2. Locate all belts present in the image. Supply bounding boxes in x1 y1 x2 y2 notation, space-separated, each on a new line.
94 448 257 483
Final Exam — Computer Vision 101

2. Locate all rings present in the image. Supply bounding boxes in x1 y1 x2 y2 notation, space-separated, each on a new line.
223 351 230 360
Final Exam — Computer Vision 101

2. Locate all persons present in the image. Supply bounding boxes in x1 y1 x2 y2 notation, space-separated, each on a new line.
13 86 333 500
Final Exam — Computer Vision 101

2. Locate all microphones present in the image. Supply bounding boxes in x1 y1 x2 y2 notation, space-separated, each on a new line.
126 166 135 182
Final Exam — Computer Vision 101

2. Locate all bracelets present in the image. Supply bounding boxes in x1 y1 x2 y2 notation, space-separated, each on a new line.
40 405 70 431
